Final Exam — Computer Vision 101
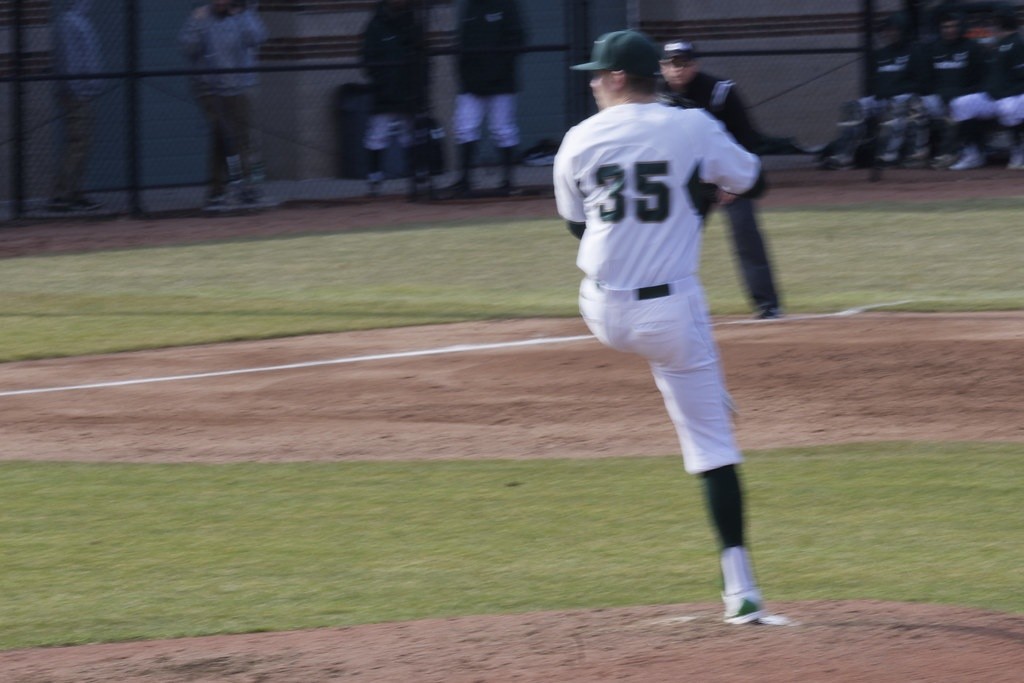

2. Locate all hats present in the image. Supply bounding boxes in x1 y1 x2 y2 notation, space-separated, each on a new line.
569 29 662 79
659 38 698 60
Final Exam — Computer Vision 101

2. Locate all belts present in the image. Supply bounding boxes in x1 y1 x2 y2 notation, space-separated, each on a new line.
587 276 698 302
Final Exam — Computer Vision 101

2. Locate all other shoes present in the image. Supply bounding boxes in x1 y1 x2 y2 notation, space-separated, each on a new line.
1006 145 1024 170
930 153 959 169
949 148 986 170
827 155 859 171
875 153 902 169
365 178 525 201
755 304 779 319
904 153 930 168
204 191 277 210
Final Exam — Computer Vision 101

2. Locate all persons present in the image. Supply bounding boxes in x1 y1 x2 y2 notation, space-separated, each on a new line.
937 19 1023 170
876 16 977 169
814 12 919 171
351 0 443 195
437 1 524 196
553 31 782 628
39 4 115 219
646 35 786 318
181 1 271 205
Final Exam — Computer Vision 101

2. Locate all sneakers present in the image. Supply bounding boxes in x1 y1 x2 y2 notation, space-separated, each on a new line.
46 196 103 210
719 589 767 625
